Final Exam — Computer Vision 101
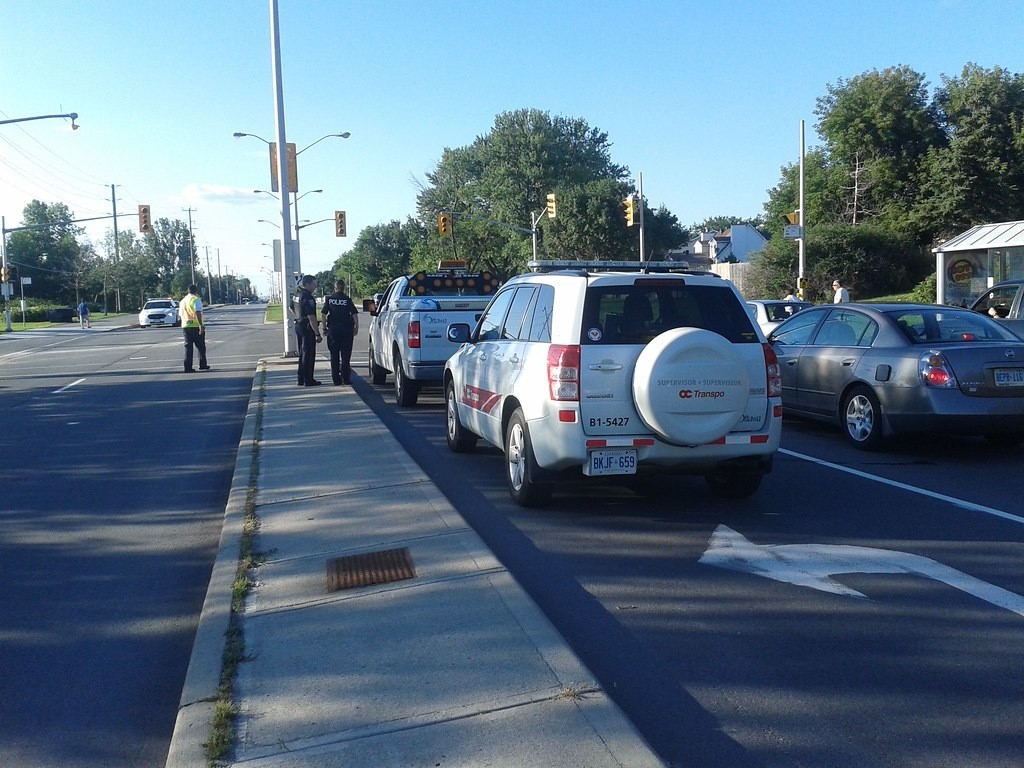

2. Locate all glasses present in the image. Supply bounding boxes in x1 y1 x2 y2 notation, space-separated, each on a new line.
832 285 837 286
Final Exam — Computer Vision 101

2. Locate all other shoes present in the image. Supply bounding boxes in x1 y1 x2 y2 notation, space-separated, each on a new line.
298 381 303 385
185 369 194 372
199 366 210 369
344 380 353 384
335 380 342 385
305 381 321 385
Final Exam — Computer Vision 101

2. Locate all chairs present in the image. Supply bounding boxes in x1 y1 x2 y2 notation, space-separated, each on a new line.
621 294 653 334
822 324 856 346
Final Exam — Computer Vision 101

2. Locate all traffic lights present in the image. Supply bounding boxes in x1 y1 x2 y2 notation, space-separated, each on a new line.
623 194 633 227
138 204 151 232
782 213 795 226
545 194 556 219
10 266 18 281
335 211 346 237
438 213 451 236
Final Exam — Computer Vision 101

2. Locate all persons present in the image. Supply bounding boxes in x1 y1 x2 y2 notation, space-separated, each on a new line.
988 307 1000 318
78 299 90 329
289 275 323 387
321 280 358 386
180 284 210 372
833 279 850 304
783 288 801 315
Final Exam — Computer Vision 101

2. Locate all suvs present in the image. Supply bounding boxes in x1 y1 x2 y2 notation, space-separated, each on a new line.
443 259 780 510
373 293 384 305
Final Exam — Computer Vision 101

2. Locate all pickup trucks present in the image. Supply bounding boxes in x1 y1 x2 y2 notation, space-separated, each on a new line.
363 259 504 407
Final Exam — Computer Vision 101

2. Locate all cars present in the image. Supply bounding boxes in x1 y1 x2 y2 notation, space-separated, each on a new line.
244 298 270 305
174 300 181 327
137 297 178 328
764 301 1024 450
911 278 1024 345
744 298 814 338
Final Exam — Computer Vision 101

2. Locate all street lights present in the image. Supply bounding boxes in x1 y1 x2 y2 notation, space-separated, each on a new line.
232 132 351 304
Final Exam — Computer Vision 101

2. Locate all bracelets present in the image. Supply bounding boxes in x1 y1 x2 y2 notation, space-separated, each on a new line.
993 313 997 316
323 328 327 330
316 334 320 336
